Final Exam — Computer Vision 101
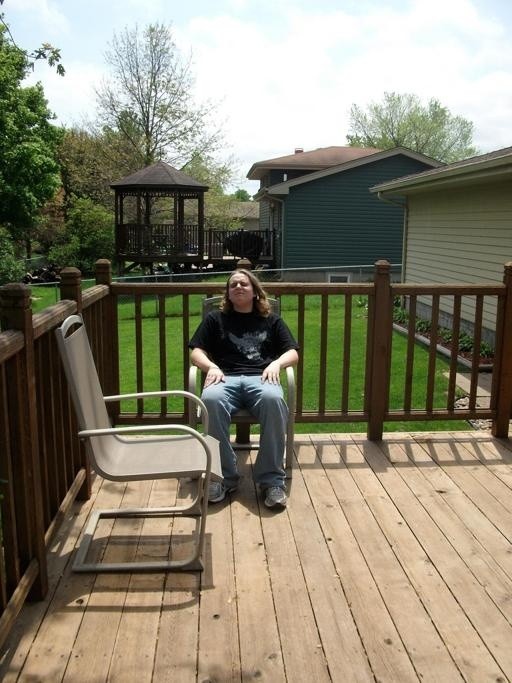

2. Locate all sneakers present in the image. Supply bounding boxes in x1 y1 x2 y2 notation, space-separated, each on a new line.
201 474 240 503
261 481 288 509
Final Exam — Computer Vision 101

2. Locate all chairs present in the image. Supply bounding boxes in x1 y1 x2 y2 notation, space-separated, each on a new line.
176 295 297 489
51 313 224 576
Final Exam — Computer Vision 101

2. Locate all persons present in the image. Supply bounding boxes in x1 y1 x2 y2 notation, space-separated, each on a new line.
189 267 300 511
